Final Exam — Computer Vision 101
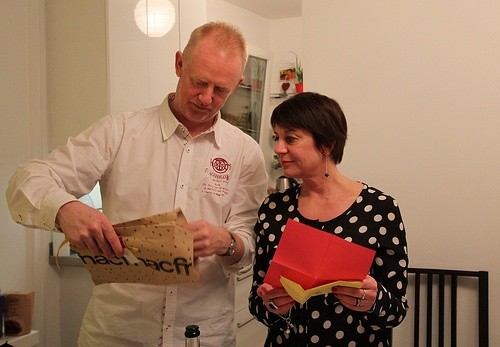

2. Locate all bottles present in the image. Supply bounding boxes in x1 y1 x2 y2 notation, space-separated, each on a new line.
184 324 200 347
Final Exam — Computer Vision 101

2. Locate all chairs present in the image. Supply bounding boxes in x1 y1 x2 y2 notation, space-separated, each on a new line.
387 267 489 347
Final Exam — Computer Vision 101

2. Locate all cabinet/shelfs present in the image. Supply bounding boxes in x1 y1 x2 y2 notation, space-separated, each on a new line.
220 55 268 143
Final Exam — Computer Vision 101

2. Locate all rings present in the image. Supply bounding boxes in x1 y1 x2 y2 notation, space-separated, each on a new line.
268 297 279 310
357 298 361 307
362 289 367 299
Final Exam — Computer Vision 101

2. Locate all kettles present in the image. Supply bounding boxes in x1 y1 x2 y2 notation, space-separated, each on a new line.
275 175 298 193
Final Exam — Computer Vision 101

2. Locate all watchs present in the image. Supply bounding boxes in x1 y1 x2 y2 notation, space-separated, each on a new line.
216 231 238 257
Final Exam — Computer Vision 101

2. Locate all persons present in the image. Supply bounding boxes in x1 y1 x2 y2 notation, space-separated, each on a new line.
5 20 269 347
249 92 409 347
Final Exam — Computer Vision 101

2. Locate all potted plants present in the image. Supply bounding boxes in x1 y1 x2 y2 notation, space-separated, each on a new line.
291 53 303 96
